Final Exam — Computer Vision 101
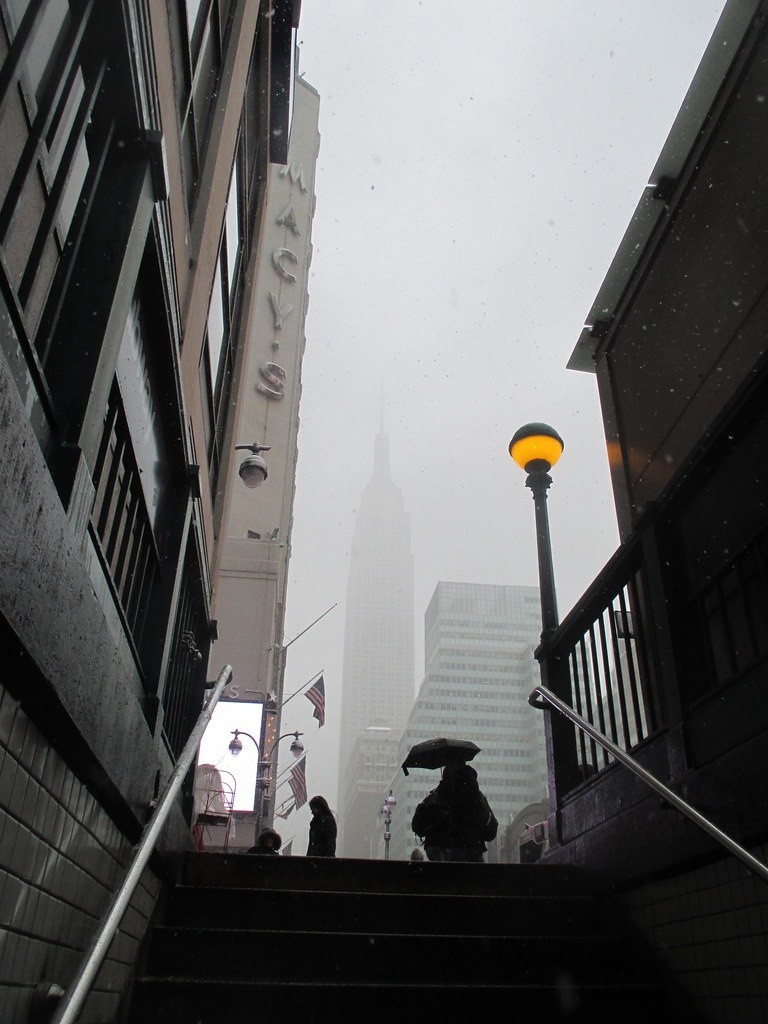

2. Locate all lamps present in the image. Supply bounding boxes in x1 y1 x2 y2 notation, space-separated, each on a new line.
266 644 280 651
235 440 271 489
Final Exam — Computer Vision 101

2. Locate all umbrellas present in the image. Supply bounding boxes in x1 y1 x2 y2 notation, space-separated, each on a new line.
402 734 481 779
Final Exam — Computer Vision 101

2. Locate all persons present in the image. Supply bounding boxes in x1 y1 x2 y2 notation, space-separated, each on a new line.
410 762 497 860
306 797 337 858
245 827 282 854
412 849 424 860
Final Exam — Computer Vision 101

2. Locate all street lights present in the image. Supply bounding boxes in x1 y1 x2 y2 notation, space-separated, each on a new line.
507 421 565 648
228 728 305 847
380 789 397 862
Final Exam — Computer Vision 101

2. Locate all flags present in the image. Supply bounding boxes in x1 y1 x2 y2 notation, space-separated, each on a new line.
289 777 307 810
291 757 306 793
277 803 294 820
305 676 324 728
282 842 292 856
